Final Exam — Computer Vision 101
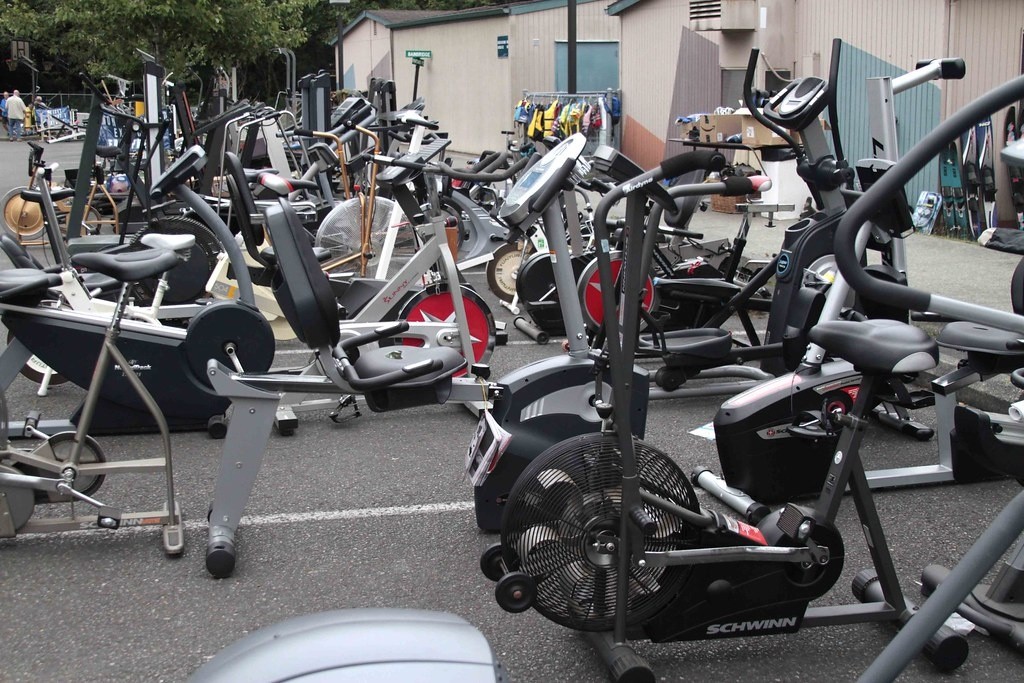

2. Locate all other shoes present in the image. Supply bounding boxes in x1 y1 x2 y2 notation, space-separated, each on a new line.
17 138 22 141
10 137 13 140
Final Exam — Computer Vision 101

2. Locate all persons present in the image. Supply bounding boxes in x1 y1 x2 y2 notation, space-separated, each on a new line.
0 90 46 143
92 86 123 164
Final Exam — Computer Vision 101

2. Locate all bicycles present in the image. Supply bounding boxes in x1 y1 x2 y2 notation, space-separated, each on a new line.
1 43 1024 683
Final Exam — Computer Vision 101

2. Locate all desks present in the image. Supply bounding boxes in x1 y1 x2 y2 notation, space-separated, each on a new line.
668 138 792 178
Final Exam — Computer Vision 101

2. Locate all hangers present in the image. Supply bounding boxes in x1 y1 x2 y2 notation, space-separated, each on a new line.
524 92 600 106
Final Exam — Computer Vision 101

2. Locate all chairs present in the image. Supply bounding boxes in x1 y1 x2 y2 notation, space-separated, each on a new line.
223 149 333 267
266 197 468 411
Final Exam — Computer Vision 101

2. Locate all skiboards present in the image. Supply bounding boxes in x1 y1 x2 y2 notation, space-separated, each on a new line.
1002 105 1024 231
937 141 968 242
961 114 999 239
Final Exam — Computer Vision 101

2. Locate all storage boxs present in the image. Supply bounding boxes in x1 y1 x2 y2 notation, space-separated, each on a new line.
707 178 761 214
678 122 700 141
700 112 741 142
733 114 791 146
